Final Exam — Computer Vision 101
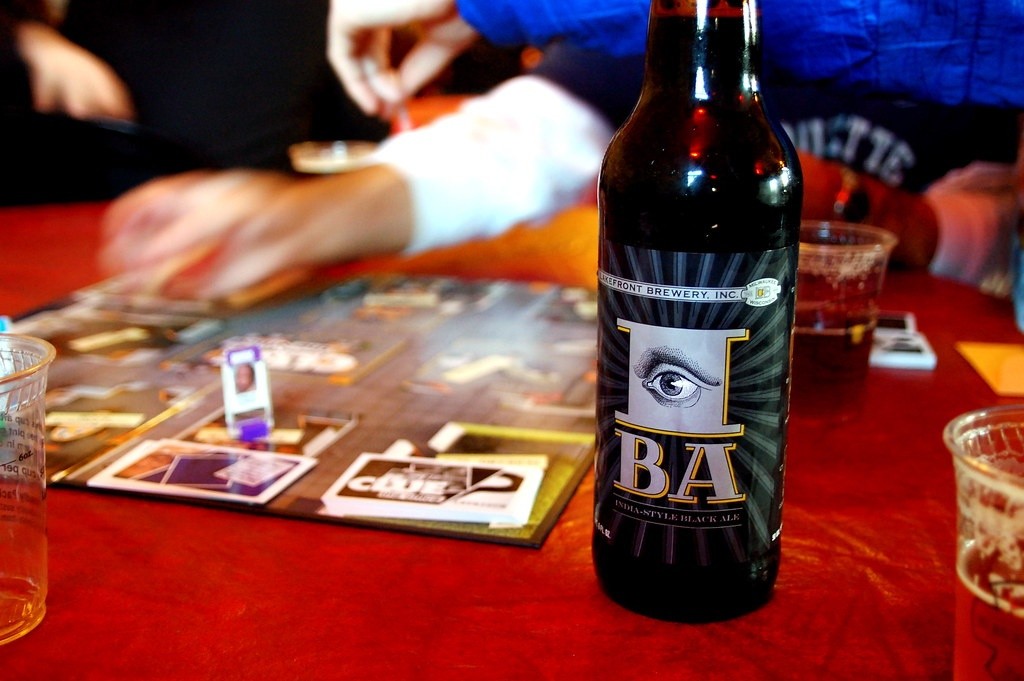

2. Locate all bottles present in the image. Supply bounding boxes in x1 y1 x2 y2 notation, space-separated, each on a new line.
593 0 802 624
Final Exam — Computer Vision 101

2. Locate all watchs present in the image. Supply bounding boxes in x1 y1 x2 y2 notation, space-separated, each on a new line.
828 167 874 225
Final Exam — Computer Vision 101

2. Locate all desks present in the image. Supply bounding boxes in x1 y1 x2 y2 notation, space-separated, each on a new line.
0 199 1024 681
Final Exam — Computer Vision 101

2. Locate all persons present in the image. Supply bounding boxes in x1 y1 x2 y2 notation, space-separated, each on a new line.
102 1 1024 301
1 0 387 207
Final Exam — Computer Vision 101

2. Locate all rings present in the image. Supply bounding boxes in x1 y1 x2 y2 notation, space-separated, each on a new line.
329 139 347 161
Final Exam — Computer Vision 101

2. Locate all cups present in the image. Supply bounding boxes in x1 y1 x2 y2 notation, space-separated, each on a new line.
0 330 56 645
943 409 1024 681
795 220 897 379
